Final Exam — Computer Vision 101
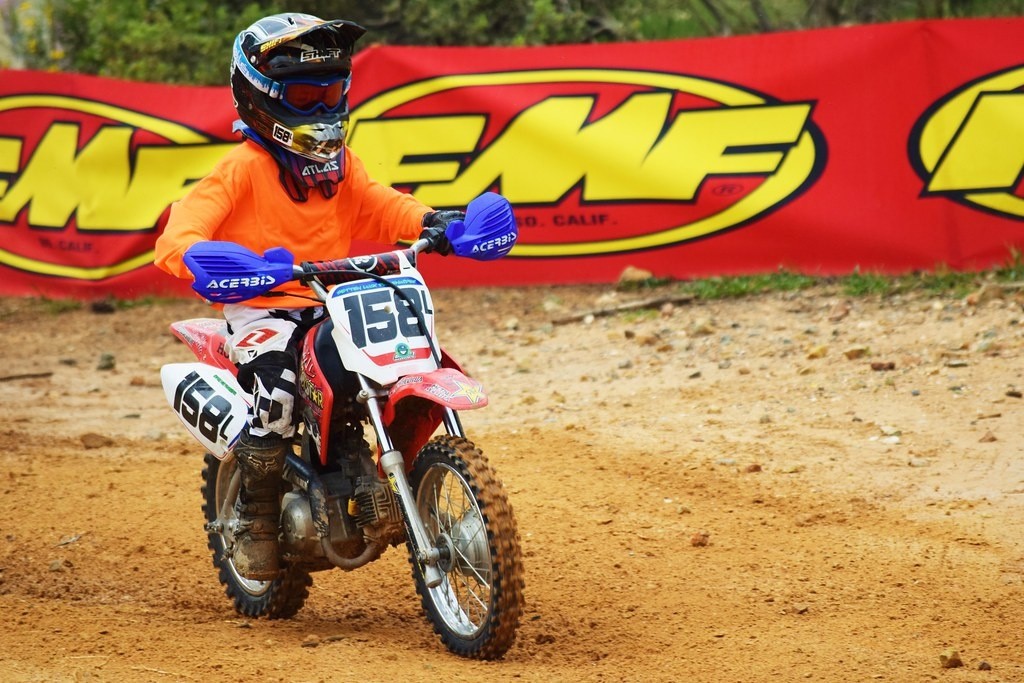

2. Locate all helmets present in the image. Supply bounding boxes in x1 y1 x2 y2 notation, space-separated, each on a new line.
230 12 367 163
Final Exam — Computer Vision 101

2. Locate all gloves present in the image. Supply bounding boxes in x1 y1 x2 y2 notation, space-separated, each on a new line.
422 210 466 232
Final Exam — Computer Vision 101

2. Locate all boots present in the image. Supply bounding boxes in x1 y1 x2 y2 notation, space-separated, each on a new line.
230 426 286 582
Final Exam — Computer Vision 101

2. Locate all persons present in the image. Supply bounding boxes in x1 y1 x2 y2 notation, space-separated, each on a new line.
152 12 468 583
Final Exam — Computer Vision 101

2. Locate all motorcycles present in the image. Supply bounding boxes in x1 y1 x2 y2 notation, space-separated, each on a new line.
159 191 526 661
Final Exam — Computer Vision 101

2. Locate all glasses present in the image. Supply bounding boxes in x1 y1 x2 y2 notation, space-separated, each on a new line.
268 76 352 114
